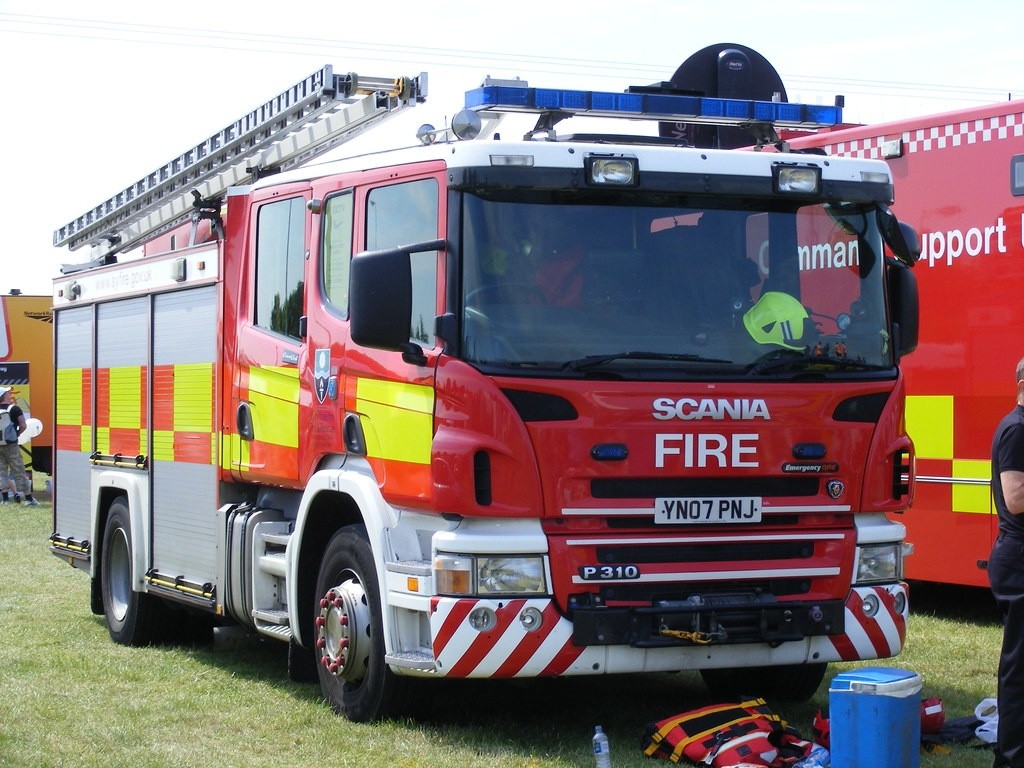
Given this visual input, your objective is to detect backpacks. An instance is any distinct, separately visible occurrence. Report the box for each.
[0,404,18,445]
[645,697,813,768]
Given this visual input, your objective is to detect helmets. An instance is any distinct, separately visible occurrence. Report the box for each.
[743,291,810,351]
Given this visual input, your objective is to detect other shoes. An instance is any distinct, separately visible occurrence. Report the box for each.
[25,497,40,506]
[2,500,9,505]
[14,494,21,504]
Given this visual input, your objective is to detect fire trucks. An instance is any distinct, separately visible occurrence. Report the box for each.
[653,100,1024,611]
[49,42,920,725]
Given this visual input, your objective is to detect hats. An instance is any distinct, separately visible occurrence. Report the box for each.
[0,387,11,397]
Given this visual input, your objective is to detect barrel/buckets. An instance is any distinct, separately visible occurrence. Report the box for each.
[829,666,922,768]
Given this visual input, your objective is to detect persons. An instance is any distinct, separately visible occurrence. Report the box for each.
[0,387,39,506]
[986,356,1024,768]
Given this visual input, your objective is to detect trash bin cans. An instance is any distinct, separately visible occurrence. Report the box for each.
[826,667,925,768]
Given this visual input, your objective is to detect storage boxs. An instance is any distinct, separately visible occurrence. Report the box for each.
[829,667,923,768]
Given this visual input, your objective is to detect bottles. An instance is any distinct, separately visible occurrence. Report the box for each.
[790,747,830,768]
[592,727,610,768]
